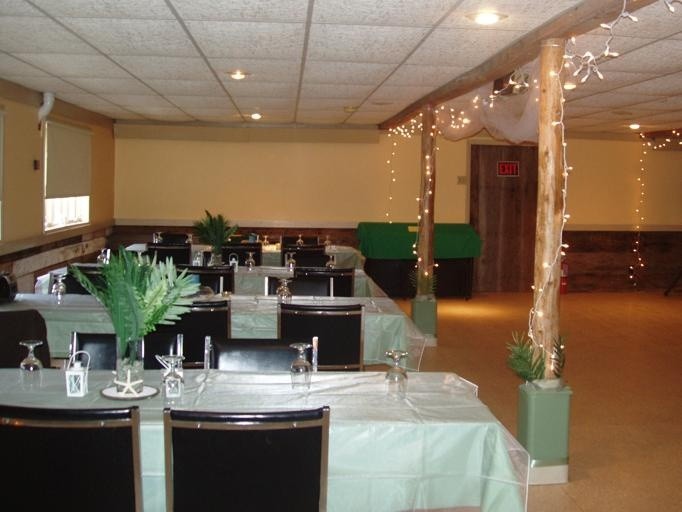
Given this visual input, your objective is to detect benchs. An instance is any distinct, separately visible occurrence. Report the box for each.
[565,250,647,291]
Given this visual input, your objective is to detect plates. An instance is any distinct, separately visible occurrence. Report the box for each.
[101,386,159,400]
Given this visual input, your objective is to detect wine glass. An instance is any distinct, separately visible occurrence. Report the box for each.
[288,342,313,389]
[51,275,69,306]
[96,230,336,271]
[17,339,45,388]
[162,355,187,406]
[382,350,408,401]
[276,278,292,305]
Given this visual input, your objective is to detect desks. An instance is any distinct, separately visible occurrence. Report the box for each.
[125,243,367,269]
[48,262,370,298]
[0,368,488,512]
[1,292,406,372]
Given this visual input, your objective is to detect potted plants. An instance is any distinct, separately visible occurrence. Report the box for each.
[188,212,238,267]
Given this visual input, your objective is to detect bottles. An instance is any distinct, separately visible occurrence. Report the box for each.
[64,360,88,398]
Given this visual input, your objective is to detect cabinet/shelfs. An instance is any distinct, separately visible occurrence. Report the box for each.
[360,221,482,301]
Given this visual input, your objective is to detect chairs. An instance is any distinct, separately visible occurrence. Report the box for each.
[280,236,335,266]
[176,264,235,295]
[1,309,59,370]
[164,405,330,511]
[65,264,114,293]
[203,333,320,373]
[222,236,263,264]
[277,302,365,372]
[65,244,206,335]
[264,274,335,297]
[147,232,193,265]
[140,301,233,369]
[3,405,144,511]
[69,329,185,367]
[290,265,358,295]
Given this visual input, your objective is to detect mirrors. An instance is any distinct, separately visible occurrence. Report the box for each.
[40,120,94,235]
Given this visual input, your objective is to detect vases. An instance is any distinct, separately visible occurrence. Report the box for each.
[115,335,143,394]
